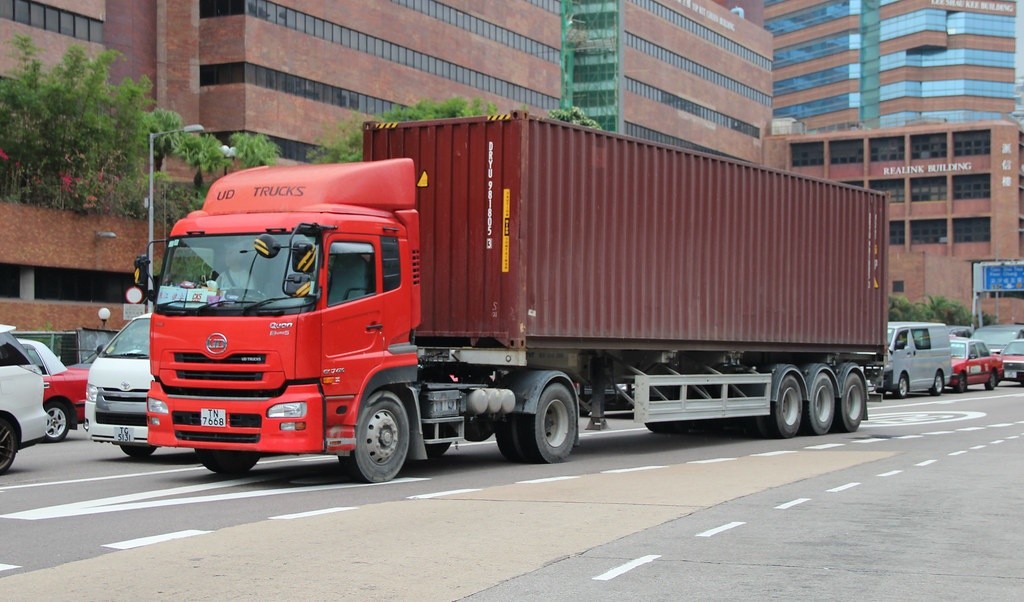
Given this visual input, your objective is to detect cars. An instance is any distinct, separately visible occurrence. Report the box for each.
[0,324,48,473]
[970,324,1024,354]
[84,312,157,459]
[17,338,98,443]
[947,325,974,339]
[998,339,1024,387]
[946,337,1003,393]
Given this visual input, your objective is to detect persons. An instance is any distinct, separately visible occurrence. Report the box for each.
[216,246,254,290]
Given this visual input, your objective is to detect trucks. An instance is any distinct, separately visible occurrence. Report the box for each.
[134,108,891,484]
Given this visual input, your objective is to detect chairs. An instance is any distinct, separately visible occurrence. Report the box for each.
[325,253,370,306]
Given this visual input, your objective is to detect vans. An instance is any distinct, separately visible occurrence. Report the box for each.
[861,322,952,399]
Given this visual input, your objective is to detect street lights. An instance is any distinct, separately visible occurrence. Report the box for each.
[148,123,204,312]
[219,145,237,176]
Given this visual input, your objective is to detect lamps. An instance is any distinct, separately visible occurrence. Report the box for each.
[97,231,116,239]
